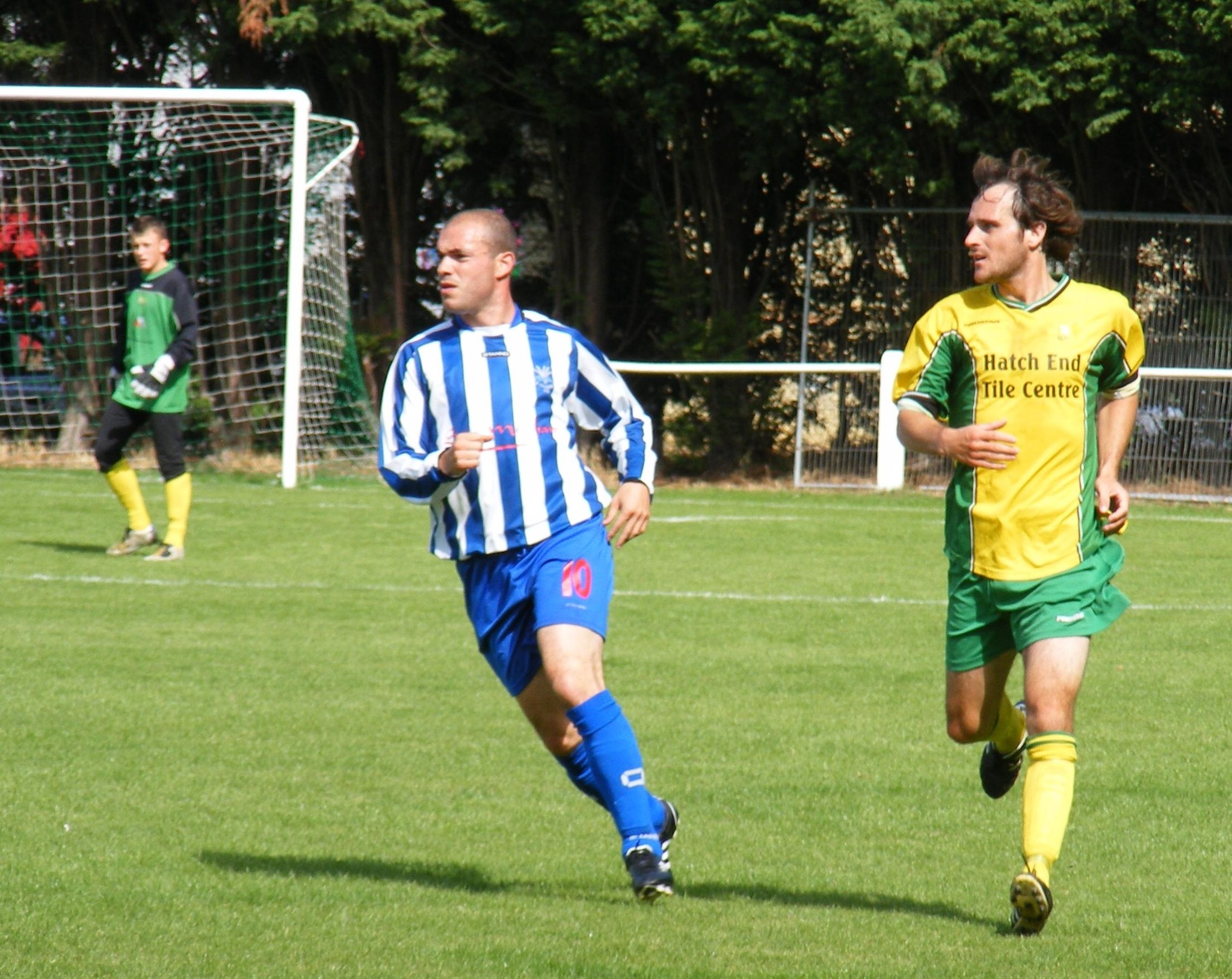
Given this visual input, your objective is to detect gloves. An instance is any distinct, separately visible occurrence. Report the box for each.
[130,353,175,399]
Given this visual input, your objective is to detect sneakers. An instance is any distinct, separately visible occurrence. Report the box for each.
[106,527,158,555]
[656,797,678,895]
[623,845,674,901]
[1009,848,1053,936]
[147,543,184,561]
[980,701,1029,798]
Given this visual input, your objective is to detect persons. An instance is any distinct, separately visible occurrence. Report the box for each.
[377,209,680,900]
[94,216,198,561]
[891,149,1146,937]
